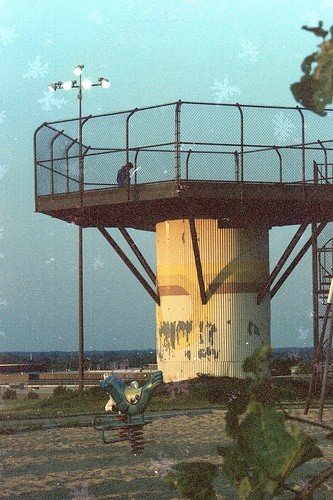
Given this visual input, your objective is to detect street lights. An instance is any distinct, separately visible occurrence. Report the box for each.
[48,65,112,389]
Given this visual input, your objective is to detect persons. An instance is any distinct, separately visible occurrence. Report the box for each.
[116,162,133,189]
